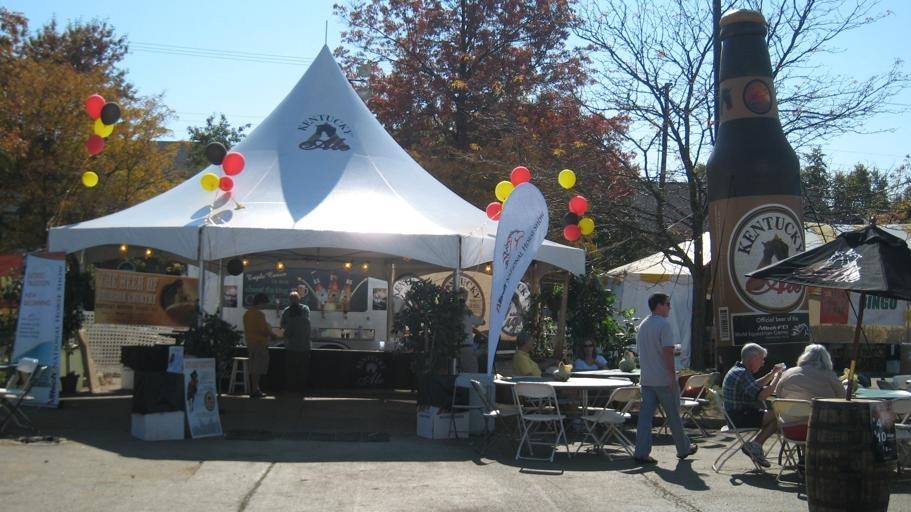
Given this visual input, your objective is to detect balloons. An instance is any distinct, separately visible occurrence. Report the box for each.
[511,166,531,187]
[95,118,114,138]
[564,213,577,224]
[83,171,98,187]
[202,174,220,191]
[559,169,576,188]
[87,135,104,155]
[486,202,502,220]
[564,225,581,241]
[86,94,105,119]
[222,153,245,175]
[220,177,233,191]
[207,142,227,164]
[569,196,588,216]
[101,103,121,124]
[578,218,594,235]
[495,180,515,202]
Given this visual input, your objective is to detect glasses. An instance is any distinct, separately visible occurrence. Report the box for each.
[584,344,593,347]
[666,302,670,306]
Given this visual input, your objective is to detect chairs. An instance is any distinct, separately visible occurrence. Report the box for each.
[708,387,765,476]
[772,398,812,487]
[575,387,639,463]
[659,373,719,440]
[577,375,630,413]
[515,381,571,463]
[448,372,492,442]
[470,379,523,454]
[0,357,47,433]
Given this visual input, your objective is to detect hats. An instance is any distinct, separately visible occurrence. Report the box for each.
[253,293,269,304]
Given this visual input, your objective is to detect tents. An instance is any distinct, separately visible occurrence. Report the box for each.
[598,222,911,370]
[47,42,584,375]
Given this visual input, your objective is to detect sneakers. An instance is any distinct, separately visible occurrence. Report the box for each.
[743,442,770,468]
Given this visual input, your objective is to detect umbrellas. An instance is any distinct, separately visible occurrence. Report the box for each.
[745,214,911,399]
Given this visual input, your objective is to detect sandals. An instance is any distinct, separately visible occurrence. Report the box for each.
[635,456,658,464]
[676,442,698,460]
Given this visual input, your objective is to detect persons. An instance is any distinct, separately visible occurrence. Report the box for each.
[283,305,312,397]
[775,343,847,440]
[574,337,609,369]
[722,342,783,467]
[634,293,697,464]
[242,293,270,399]
[280,291,310,349]
[513,333,542,377]
[190,298,199,331]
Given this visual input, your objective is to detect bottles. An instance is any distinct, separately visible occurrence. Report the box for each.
[328,271,339,310]
[340,274,354,312]
[309,269,327,310]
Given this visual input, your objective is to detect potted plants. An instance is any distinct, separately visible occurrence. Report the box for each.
[59,264,97,394]
[391,274,473,441]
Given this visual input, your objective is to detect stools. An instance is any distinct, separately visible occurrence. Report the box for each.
[228,356,249,395]
